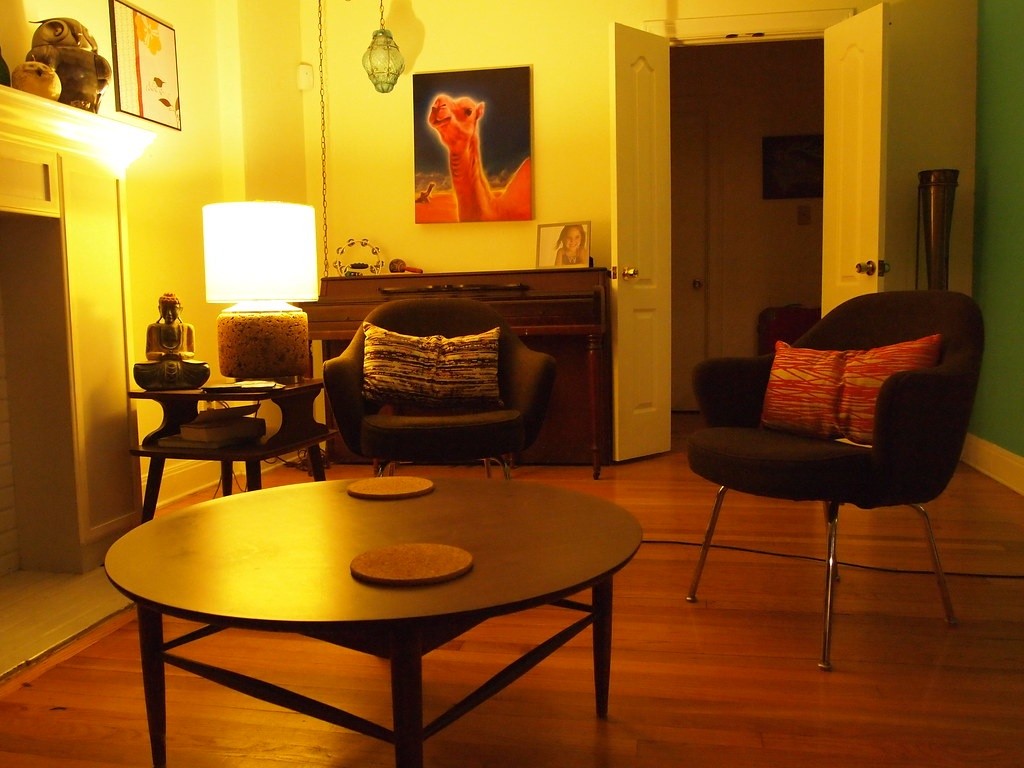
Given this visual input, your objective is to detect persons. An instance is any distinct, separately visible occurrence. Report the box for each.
[554,225,588,267]
[134,293,210,389]
[158,402,266,449]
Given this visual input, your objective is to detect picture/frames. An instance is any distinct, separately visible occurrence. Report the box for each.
[534,221,591,268]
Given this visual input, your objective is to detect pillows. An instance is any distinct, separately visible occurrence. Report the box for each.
[361,322,505,405]
[759,333,944,445]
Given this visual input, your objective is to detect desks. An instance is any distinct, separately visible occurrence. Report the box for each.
[104,477,644,768]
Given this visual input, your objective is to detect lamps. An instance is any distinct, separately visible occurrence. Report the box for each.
[362,0,405,93]
[202,200,319,386]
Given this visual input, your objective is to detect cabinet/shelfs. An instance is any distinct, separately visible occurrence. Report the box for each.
[124,375,337,526]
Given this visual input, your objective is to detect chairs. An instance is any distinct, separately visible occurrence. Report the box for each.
[684,289,986,670]
[323,298,558,478]
